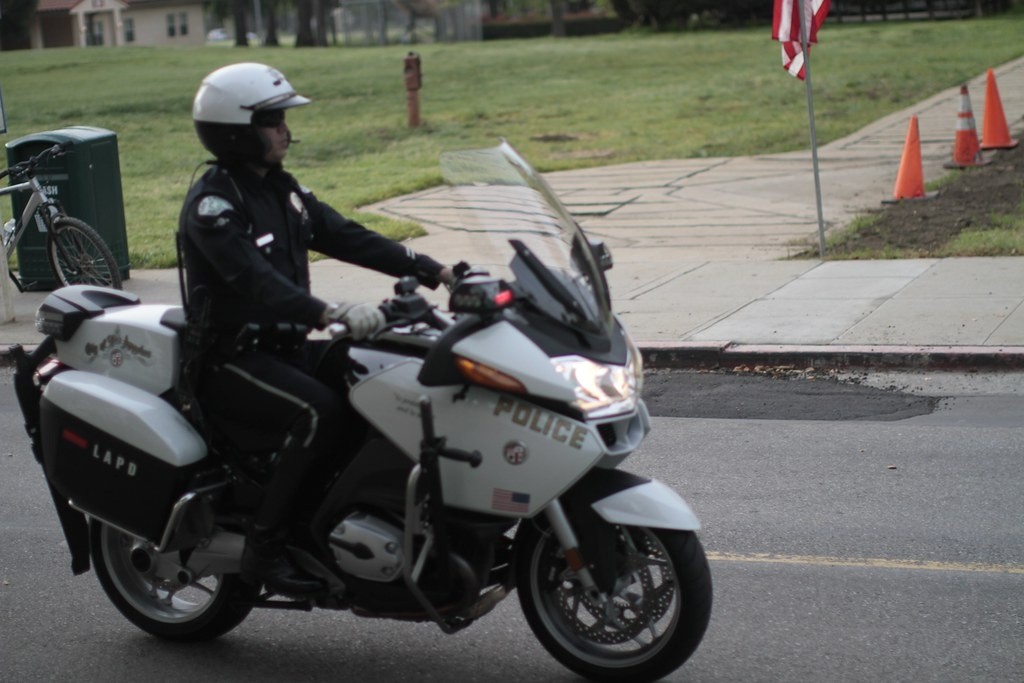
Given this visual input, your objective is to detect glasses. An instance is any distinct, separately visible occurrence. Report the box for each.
[242,109,289,129]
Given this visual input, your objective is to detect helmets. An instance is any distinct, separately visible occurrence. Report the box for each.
[190,62,314,123]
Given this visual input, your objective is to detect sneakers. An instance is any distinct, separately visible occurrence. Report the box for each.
[240,539,346,600]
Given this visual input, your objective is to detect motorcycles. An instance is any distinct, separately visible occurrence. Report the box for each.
[5,140,718,683]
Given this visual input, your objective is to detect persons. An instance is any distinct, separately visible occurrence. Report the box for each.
[174,61,474,595]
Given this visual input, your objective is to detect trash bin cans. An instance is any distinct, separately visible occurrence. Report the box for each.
[4,124,132,292]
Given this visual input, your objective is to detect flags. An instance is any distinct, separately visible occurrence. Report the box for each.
[772,1,831,79]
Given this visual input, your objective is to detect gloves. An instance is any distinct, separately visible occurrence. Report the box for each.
[435,266,482,298]
[318,300,389,344]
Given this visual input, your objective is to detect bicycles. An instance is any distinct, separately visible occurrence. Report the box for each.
[0,141,123,299]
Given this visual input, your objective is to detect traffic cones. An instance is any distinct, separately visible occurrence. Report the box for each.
[942,81,993,169]
[886,113,936,204]
[973,67,1019,152]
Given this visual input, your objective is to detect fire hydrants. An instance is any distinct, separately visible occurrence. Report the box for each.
[401,48,425,128]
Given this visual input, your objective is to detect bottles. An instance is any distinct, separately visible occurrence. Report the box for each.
[2,218,15,246]
